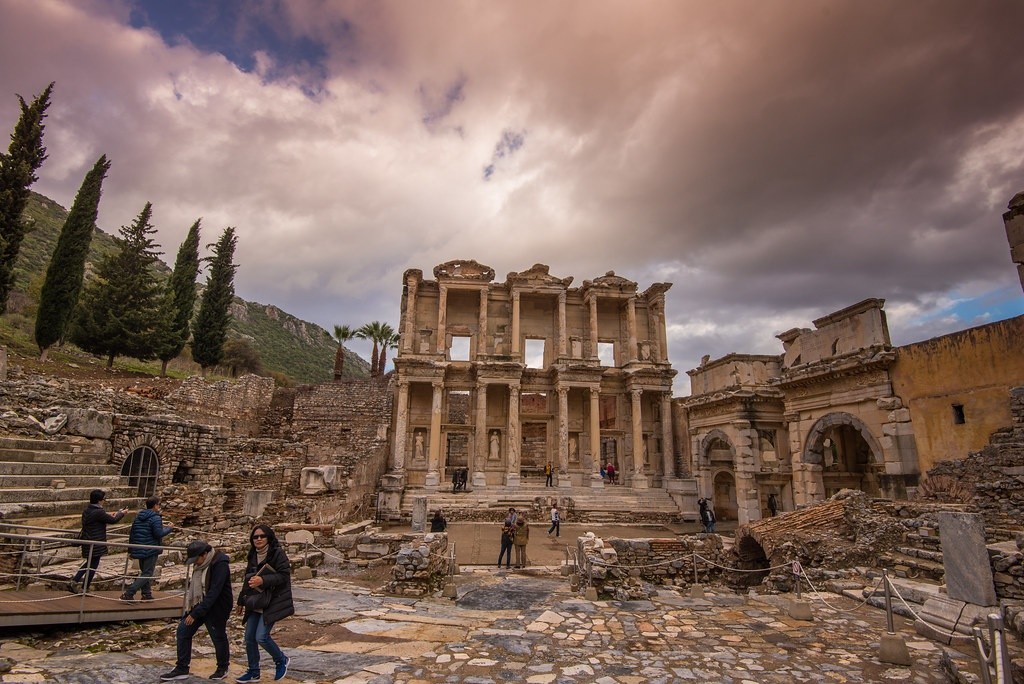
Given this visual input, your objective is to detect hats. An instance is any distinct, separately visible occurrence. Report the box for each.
[516,516,525,524]
[185,542,208,565]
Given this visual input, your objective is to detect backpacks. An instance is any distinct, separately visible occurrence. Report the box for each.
[502,528,511,545]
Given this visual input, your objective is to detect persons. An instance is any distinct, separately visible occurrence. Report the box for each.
[452,467,469,494]
[768,494,777,516]
[415,432,424,459]
[569,438,576,460]
[430,511,446,532]
[544,461,553,487]
[547,503,559,537]
[698,497,716,532]
[161,541,233,681]
[69,489,128,597]
[498,508,529,568]
[119,496,173,604]
[236,525,294,683]
[600,463,615,484]
[490,431,499,459]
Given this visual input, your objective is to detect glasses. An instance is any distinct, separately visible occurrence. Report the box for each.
[252,534,267,539]
[100,499,105,501]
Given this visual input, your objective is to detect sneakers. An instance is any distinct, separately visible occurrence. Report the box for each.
[274,656,290,681]
[209,668,228,680]
[160,666,189,681]
[235,669,260,683]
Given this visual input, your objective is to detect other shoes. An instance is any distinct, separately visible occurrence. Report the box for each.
[78,588,94,595]
[140,596,155,603]
[506,566,511,569]
[498,565,501,568]
[557,536,562,537]
[119,593,140,605]
[514,566,520,568]
[67,583,77,594]
[550,485,553,487]
[546,485,548,487]
[547,531,550,536]
[523,566,525,568]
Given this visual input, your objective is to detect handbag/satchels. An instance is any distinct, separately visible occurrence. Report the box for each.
[71,536,81,547]
[244,588,272,610]
